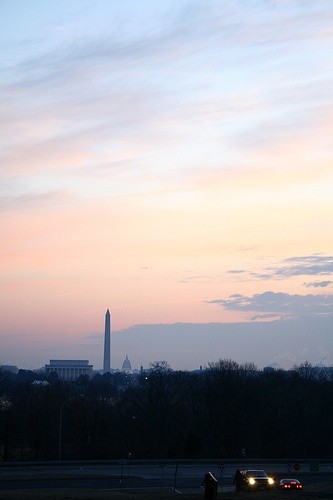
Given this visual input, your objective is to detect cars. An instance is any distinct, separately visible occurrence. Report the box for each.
[239,470,275,492]
[277,479,303,493]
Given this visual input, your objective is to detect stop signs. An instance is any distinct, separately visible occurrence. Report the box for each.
[217,462,225,469]
